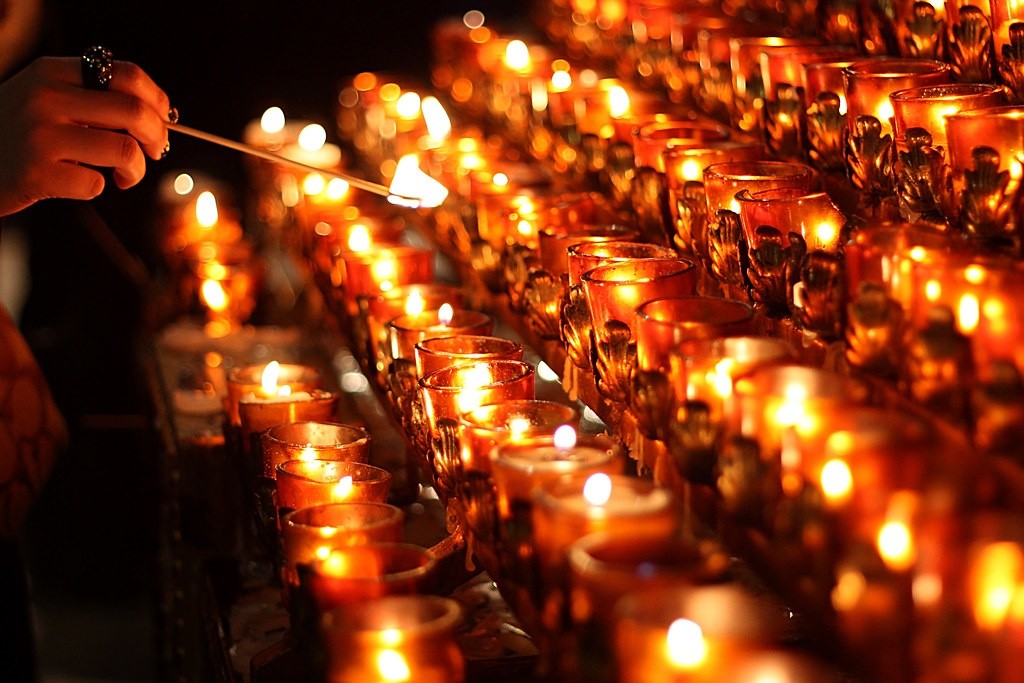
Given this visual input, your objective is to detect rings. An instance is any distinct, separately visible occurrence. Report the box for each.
[79,45,112,90]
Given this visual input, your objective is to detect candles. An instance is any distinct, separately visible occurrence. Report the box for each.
[159,0,1024,683]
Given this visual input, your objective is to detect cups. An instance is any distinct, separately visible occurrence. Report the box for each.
[136,0,1024,683]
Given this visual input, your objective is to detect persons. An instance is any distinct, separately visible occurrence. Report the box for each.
[0,0,179,683]
